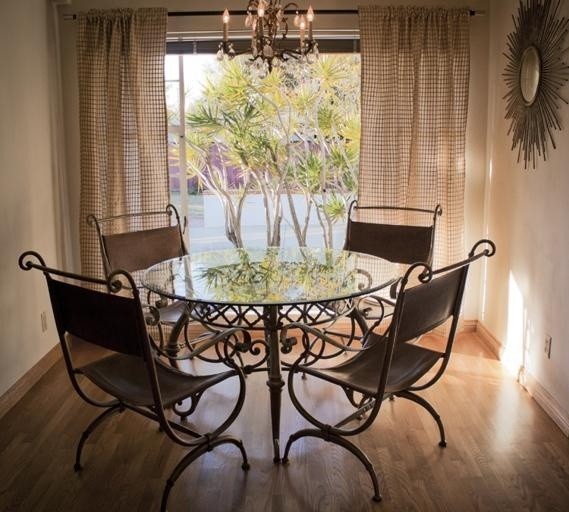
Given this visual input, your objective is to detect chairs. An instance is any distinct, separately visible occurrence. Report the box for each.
[82,203,245,405]
[20,251,249,511]
[281,238,497,502]
[299,199,443,418]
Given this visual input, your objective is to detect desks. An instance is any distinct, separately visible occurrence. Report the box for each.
[141,248,403,464]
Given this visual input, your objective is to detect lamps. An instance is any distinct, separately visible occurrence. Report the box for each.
[218,0,322,72]
